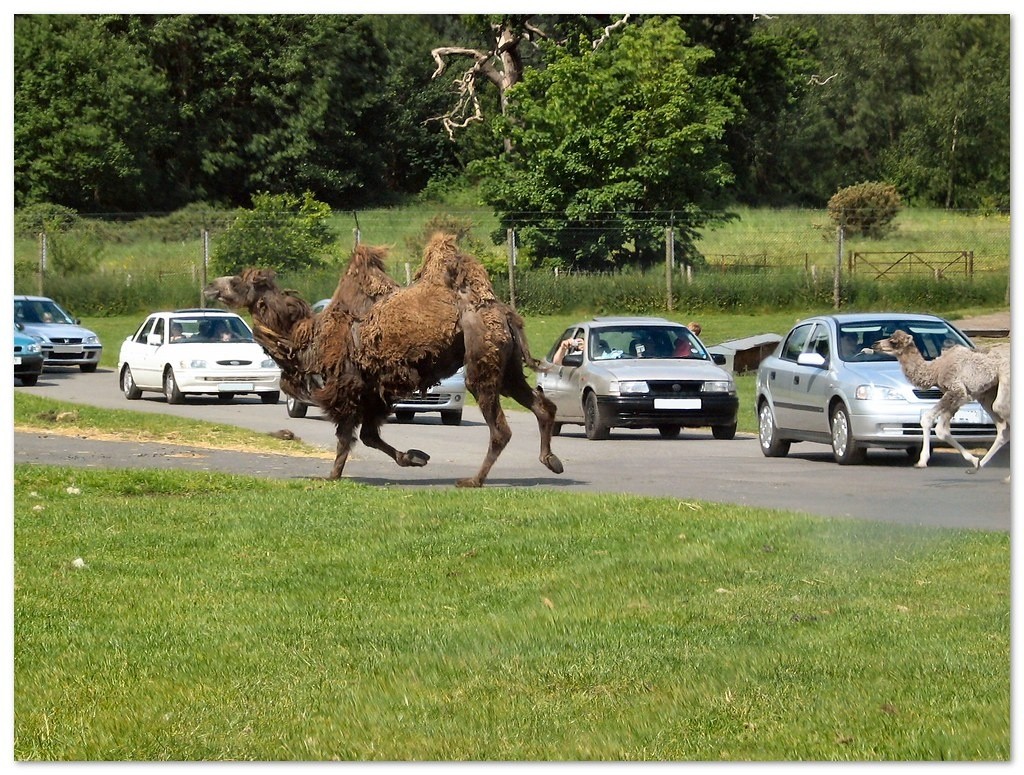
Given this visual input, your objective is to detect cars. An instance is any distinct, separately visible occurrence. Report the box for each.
[537,316,738,440]
[14,324,43,383]
[118,310,283,404]
[14,296,102,373]
[285,298,467,423]
[754,313,999,465]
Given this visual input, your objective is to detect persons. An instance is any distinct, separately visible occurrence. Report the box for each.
[839,332,873,358]
[41,312,53,323]
[171,323,186,341]
[220,329,232,341]
[674,322,701,357]
[552,337,611,369]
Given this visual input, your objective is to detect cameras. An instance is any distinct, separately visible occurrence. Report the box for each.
[571,340,579,347]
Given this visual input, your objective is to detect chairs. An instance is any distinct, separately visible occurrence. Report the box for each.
[630,339,655,358]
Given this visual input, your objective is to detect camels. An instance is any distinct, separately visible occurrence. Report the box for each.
[871,329,1010,485]
[205,233,564,487]
